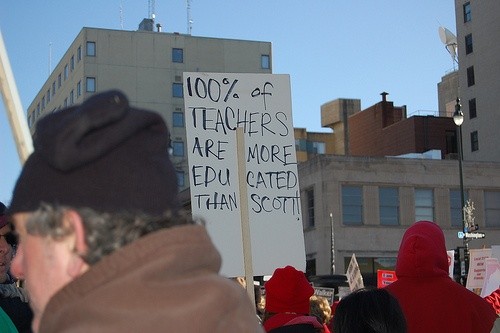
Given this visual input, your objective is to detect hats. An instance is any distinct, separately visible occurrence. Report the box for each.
[265,265,315,315]
[0,202,9,228]
[7,88,182,213]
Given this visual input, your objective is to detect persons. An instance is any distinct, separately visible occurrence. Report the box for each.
[332,287,407,333]
[8,88,264,333]
[380,221,500,333]
[0,202,35,333]
[254,266,332,333]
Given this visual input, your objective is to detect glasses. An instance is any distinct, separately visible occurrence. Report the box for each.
[0,231,17,245]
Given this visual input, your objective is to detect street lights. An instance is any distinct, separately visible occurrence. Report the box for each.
[453,96,469,273]
